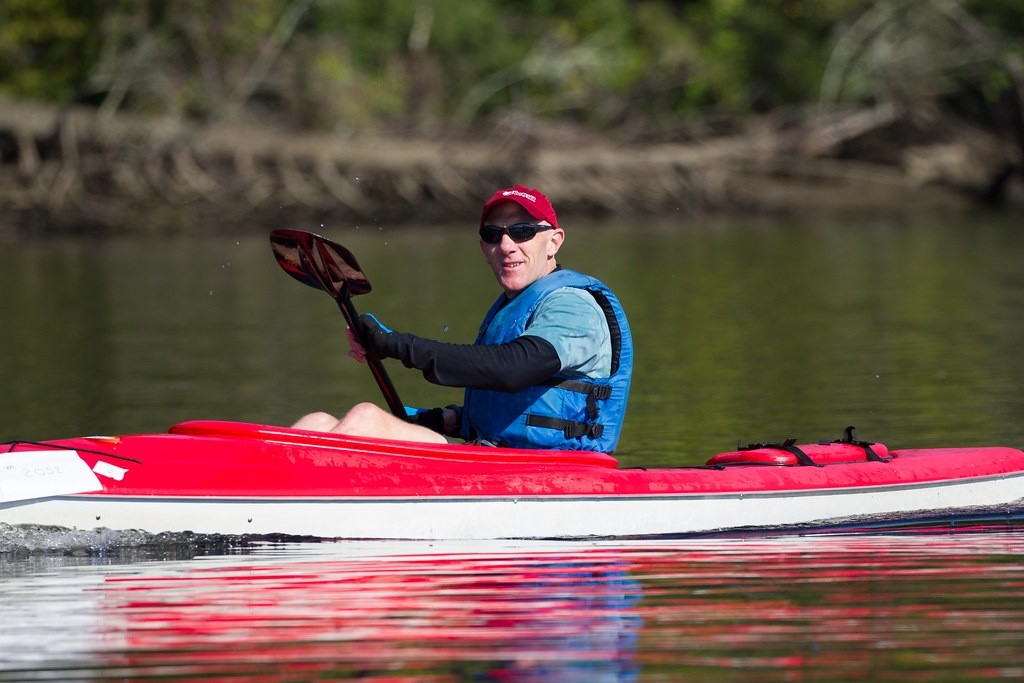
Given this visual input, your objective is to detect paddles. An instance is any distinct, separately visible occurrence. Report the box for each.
[265,225,417,423]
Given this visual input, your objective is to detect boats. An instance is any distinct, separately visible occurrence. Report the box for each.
[0,420,1024,546]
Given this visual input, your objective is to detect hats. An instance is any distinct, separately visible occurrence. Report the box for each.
[480,184,559,228]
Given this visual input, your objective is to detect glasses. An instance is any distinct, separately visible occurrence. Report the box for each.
[479,222,553,244]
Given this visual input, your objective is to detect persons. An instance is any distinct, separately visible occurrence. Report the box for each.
[286,183,634,456]
